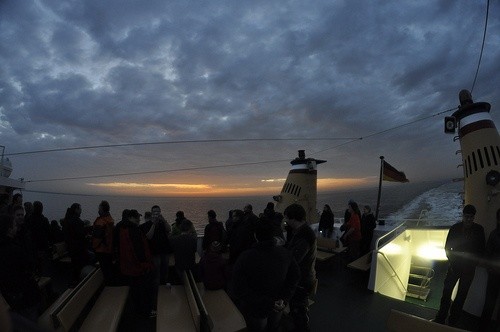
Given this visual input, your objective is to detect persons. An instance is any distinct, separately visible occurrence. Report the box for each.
[0,193,376,332]
[480,209,500,332]
[429,204,486,327]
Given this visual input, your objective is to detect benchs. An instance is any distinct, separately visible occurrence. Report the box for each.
[34,267,128,332]
[155,269,247,332]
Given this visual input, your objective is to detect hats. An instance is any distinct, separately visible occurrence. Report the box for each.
[128,210,142,218]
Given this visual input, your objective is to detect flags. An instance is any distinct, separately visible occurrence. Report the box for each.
[380,159,409,183]
